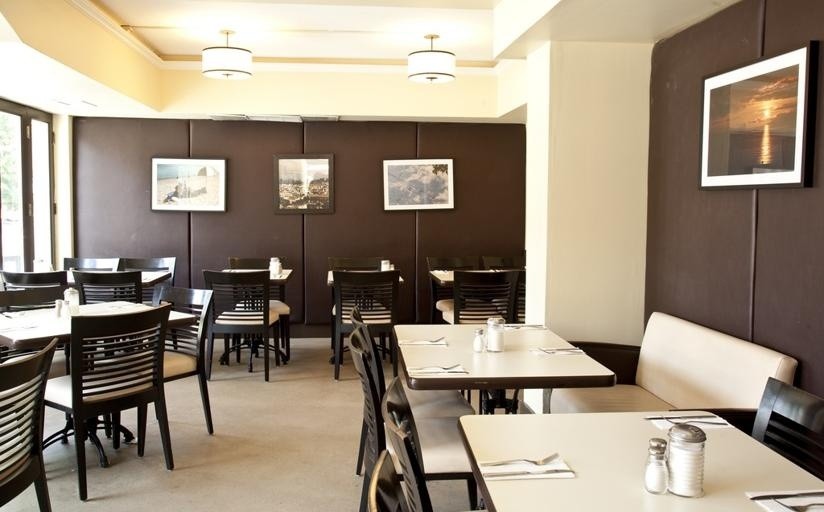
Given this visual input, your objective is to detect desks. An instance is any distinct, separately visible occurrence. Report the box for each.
[1,300,197,468]
[392,324,617,414]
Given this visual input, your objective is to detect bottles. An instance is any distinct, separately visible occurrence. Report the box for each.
[472,316,506,352]
[266,256,284,277]
[380,260,393,272]
[53,287,81,318]
[646,425,705,496]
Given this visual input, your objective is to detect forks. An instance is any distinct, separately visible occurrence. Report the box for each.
[481,454,562,466]
[773,498,824,512]
[398,336,449,343]
[660,417,728,426]
[406,364,461,370]
[540,350,585,355]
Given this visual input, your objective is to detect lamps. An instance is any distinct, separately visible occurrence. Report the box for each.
[200,27,253,82]
[407,33,456,84]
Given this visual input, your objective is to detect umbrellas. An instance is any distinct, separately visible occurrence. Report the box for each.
[198,165,218,191]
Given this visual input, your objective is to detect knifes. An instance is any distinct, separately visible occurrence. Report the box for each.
[508,324,546,331]
[750,491,822,501]
[643,415,719,420]
[535,347,580,350]
[398,343,448,346]
[483,469,575,478]
[408,371,472,376]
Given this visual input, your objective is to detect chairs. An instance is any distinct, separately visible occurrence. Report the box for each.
[1,336,59,512]
[110,285,215,457]
[382,375,478,512]
[751,377,823,480]
[366,449,411,511]
[425,253,525,324]
[457,411,823,511]
[44,303,175,503]
[348,325,479,511]
[200,256,297,383]
[2,247,178,317]
[326,254,405,381]
[349,305,475,476]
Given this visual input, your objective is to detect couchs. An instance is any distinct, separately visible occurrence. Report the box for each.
[521,311,797,414]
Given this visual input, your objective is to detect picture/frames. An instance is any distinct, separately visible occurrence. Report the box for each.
[378,156,457,212]
[697,39,816,189]
[149,154,229,213]
[273,152,335,215]
